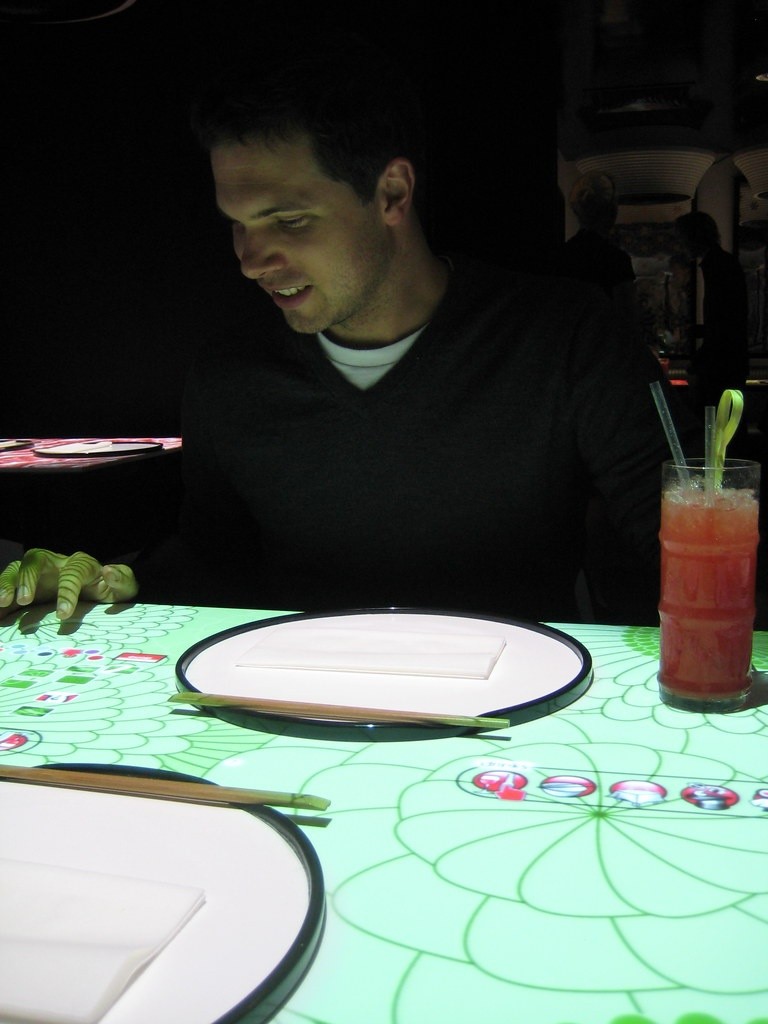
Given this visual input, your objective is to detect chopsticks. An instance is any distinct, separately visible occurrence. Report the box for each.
[0,764,331,811]
[168,692,510,728]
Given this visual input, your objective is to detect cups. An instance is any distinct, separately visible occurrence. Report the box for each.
[659,458,761,713]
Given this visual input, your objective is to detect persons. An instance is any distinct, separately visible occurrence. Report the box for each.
[0,28,681,620]
[551,161,748,432]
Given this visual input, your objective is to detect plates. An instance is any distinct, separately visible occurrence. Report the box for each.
[0,764,327,1024]
[176,604,593,745]
[0,440,34,452]
[32,442,163,457]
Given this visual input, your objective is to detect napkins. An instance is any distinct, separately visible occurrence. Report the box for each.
[0,858,205,1024]
[236,637,507,679]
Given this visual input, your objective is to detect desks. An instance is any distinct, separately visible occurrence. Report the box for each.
[0,437,184,557]
[0,607,768,1024]
